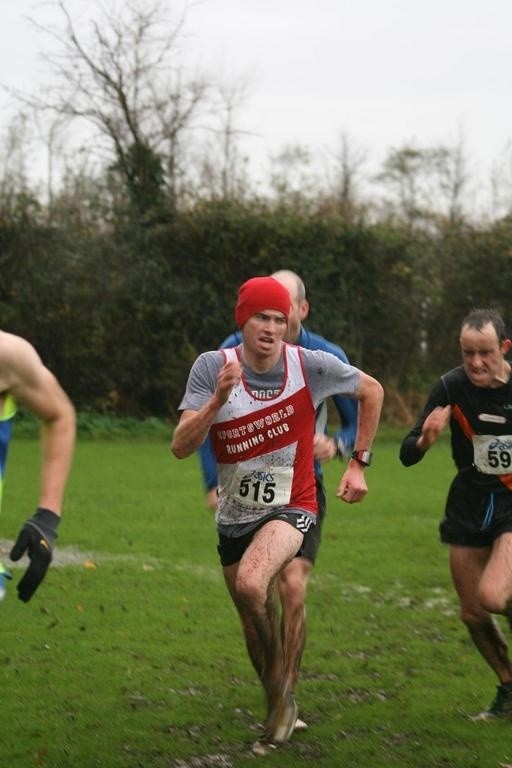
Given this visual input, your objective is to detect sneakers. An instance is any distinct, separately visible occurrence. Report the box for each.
[484,682,512,717]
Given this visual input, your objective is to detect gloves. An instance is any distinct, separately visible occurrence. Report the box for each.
[10,507,60,603]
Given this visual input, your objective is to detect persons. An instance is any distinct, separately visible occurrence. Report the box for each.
[0,329,77,602]
[400,309,512,719]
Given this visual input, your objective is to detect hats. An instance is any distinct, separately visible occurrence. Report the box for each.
[236,276,292,334]
